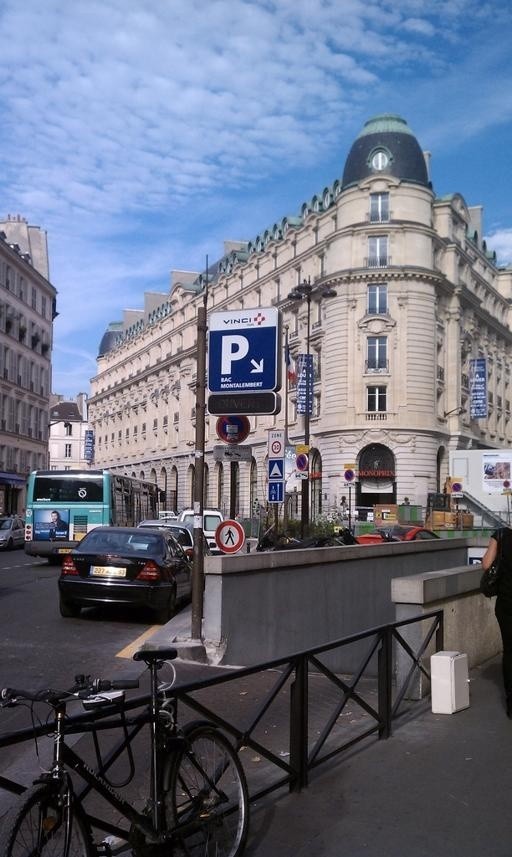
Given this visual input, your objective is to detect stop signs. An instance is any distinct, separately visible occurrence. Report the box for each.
[344,469,355,482]
[503,481,510,488]
[452,482,462,493]
[295,454,309,472]
[215,415,250,444]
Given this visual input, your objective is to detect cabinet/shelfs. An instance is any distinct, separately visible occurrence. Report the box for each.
[430,650,471,714]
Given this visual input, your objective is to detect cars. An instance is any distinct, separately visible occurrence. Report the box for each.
[355,524,443,544]
[159,510,179,523]
[57,526,193,624]
[0,517,25,551]
[127,519,216,564]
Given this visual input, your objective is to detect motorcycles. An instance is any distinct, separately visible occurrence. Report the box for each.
[255,525,361,554]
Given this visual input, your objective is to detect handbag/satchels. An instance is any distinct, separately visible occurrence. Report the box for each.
[480,565,500,598]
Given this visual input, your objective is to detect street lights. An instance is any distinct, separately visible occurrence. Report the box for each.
[287,274,337,529]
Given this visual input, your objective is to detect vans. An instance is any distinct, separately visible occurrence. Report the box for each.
[177,507,225,554]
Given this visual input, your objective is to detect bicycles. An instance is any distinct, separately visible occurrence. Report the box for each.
[0,646,251,857]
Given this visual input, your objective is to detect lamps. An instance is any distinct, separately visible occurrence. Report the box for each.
[47,419,71,428]
[444,406,467,417]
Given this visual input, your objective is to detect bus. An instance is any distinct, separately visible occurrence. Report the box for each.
[24,468,166,564]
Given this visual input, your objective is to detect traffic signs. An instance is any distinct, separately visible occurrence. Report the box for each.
[295,352,313,414]
[468,357,487,418]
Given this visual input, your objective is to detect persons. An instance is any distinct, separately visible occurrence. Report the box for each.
[402,497,410,505]
[480,526,512,719]
[443,475,453,509]
[48,511,68,543]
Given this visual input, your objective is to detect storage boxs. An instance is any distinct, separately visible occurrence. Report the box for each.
[426,493,474,528]
